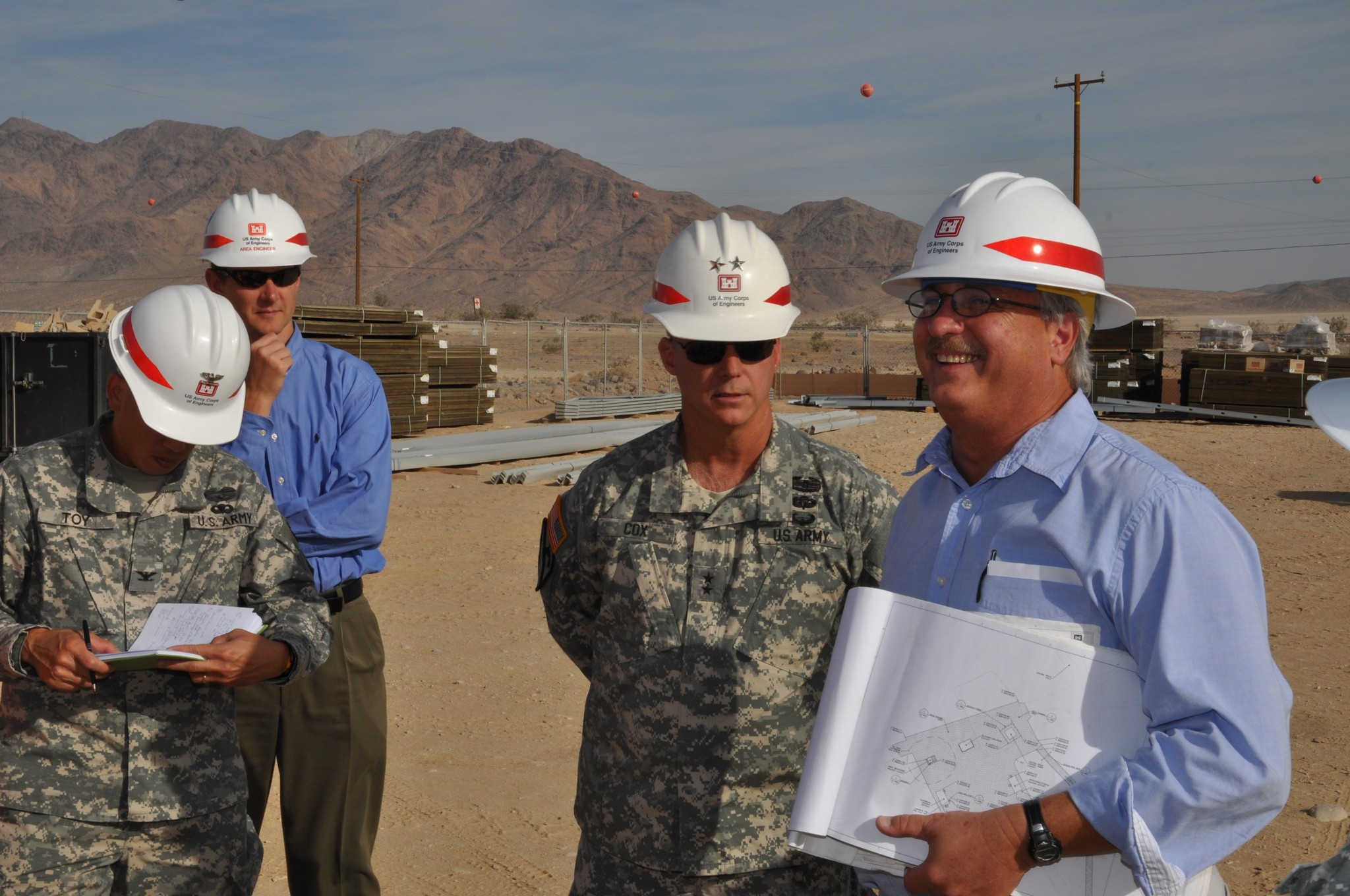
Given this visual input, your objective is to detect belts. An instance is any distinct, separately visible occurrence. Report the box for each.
[321,576,363,613]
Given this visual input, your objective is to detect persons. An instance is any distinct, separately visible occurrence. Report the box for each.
[200,188,391,896]
[538,212,898,896]
[880,171,1293,896]
[0,285,332,896]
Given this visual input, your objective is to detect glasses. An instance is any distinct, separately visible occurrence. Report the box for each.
[211,263,301,288]
[905,286,1044,318]
[672,339,777,365]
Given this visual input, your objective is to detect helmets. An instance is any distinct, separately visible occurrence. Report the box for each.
[881,171,1136,330]
[1305,376,1349,449]
[197,187,318,268]
[110,283,249,445]
[644,211,802,342]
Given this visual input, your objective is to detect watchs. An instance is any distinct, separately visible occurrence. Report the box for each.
[283,650,295,677]
[1023,798,1063,866]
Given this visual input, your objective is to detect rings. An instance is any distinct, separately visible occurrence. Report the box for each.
[202,674,208,684]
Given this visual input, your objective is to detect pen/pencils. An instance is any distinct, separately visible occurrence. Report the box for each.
[976,549,997,603]
[82,619,97,692]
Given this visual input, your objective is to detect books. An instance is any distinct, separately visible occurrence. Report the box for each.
[788,587,1153,896]
[95,602,268,671]
